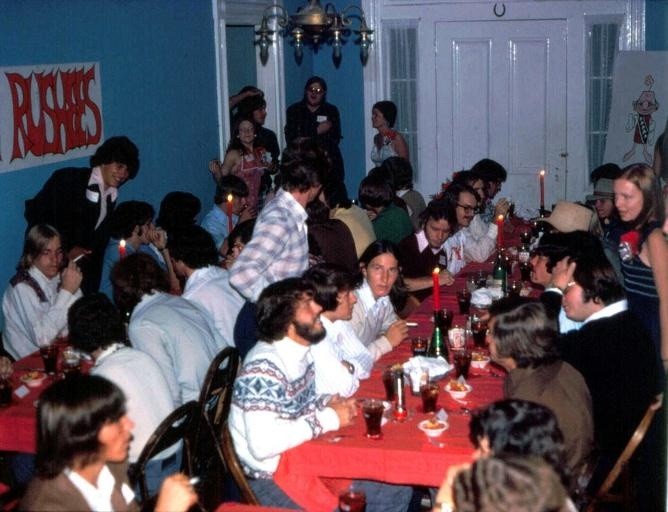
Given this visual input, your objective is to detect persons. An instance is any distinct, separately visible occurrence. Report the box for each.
[540,227,665,444]
[439,180,481,276]
[23,135,140,295]
[370,101,410,166]
[301,263,374,398]
[470,159,507,224]
[613,162,667,383]
[528,231,561,289]
[486,296,597,493]
[225,218,256,269]
[208,116,269,217]
[97,200,182,308]
[19,374,198,511]
[225,276,413,512]
[368,167,409,216]
[431,452,571,511]
[165,224,248,377]
[661,184,668,235]
[584,178,629,248]
[317,175,379,266]
[238,95,281,215]
[229,134,335,365]
[653,118,668,178]
[154,191,202,293]
[358,177,414,244]
[1,357,14,380]
[453,170,511,265]
[200,175,257,267]
[228,85,265,133]
[591,163,622,191]
[468,398,585,511]
[11,291,186,504]
[305,197,359,274]
[347,239,410,365]
[380,156,427,232]
[1,224,84,361]
[110,251,230,422]
[622,73,659,163]
[397,198,458,301]
[284,76,345,181]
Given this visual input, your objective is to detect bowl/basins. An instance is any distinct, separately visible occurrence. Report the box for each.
[417,418,448,436]
[472,353,490,369]
[21,372,46,387]
[444,383,473,399]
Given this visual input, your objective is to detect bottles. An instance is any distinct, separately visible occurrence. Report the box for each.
[493,249,507,294]
[428,312,450,362]
[539,209,548,238]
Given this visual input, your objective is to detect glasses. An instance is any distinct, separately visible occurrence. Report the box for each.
[306,85,322,94]
[562,280,578,296]
[239,125,255,133]
[455,202,482,215]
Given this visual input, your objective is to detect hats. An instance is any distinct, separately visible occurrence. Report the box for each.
[532,201,595,233]
[586,178,617,202]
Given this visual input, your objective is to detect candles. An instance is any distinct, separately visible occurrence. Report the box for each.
[433,267,440,314]
[119,239,126,258]
[227,194,232,232]
[540,170,545,206]
[497,215,502,247]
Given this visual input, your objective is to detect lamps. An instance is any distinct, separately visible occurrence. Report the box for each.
[255,0,375,69]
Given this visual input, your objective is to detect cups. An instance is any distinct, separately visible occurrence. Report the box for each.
[440,309,453,336]
[473,322,486,347]
[458,290,472,313]
[339,488,366,511]
[412,336,428,357]
[362,398,384,439]
[421,384,439,415]
[454,351,472,379]
[40,344,58,376]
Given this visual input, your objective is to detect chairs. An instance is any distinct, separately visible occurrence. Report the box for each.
[129,400,209,512]
[196,345,239,442]
[581,393,663,512]
[220,422,260,505]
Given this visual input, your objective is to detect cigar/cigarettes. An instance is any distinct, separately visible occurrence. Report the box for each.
[72,253,85,263]
[406,322,418,326]
[189,476,200,484]
